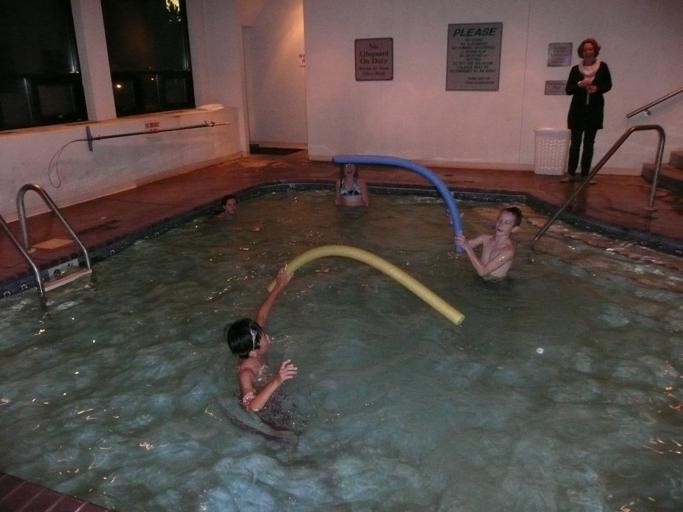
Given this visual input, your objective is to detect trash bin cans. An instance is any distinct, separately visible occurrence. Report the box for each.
[533,127,571,175]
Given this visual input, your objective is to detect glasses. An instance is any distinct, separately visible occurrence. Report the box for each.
[249,319,259,352]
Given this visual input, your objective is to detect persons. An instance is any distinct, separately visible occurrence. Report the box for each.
[560,38,611,183]
[331,165,370,209]
[226,263,301,429]
[216,196,265,232]
[454,207,521,282]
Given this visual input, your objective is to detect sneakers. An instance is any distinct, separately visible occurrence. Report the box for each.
[561,172,576,181]
[579,177,596,185]
